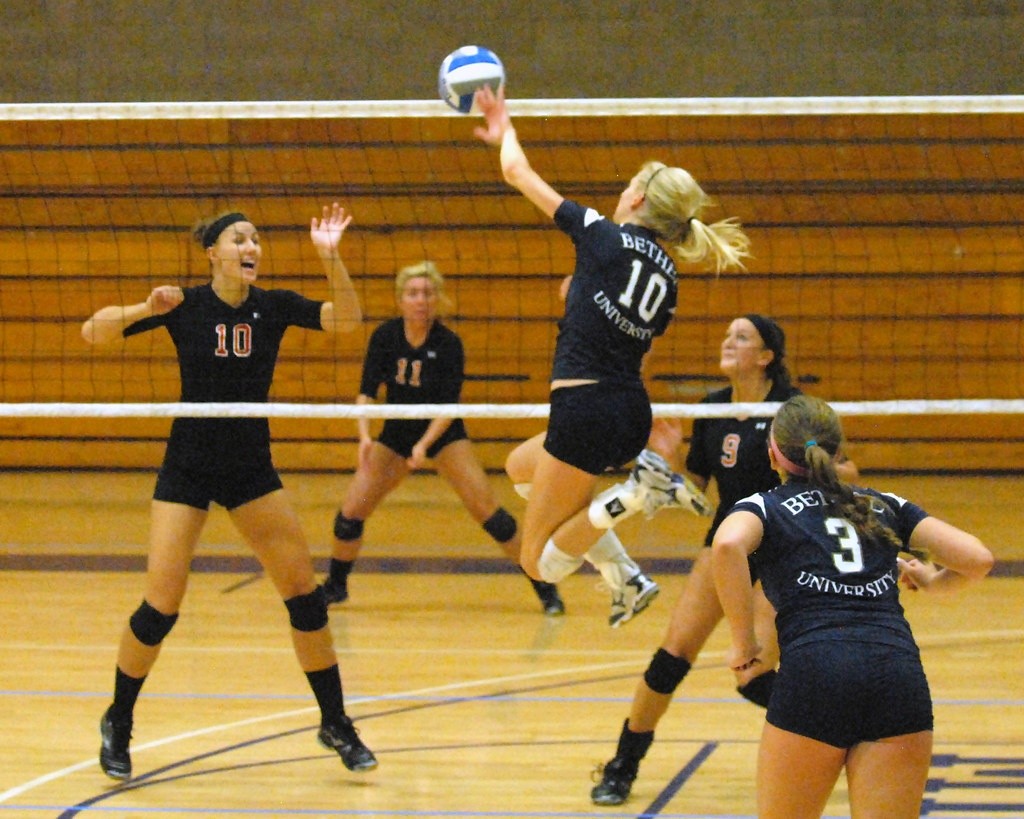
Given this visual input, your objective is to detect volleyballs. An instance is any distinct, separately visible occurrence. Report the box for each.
[435,43,509,115]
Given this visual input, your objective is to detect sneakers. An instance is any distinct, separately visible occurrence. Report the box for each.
[631,447,714,519]
[317,714,379,771]
[590,759,637,806]
[318,585,348,604]
[608,562,659,627]
[100,706,132,781]
[532,578,564,616]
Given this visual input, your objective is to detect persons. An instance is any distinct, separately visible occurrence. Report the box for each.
[472,82,753,630]
[321,261,565,617]
[710,393,994,819]
[590,312,858,806]
[80,201,379,781]
